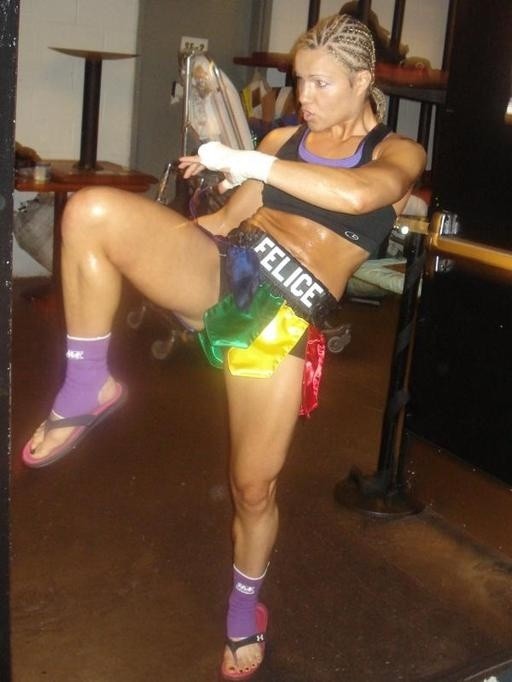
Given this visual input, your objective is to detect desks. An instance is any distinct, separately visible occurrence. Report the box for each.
[13,161,150,309]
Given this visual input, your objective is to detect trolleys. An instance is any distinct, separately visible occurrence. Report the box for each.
[129,161,354,361]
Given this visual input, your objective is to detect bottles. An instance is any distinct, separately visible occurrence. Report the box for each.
[34,161,52,182]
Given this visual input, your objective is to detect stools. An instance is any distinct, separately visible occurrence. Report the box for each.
[48,42,158,182]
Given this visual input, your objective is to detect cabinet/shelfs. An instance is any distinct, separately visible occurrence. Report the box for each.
[233,0,454,297]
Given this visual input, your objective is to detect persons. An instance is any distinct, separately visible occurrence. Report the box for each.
[18,8,432,681]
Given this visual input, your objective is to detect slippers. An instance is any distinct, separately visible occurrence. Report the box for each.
[220,602,268,680]
[22,375,129,470]
